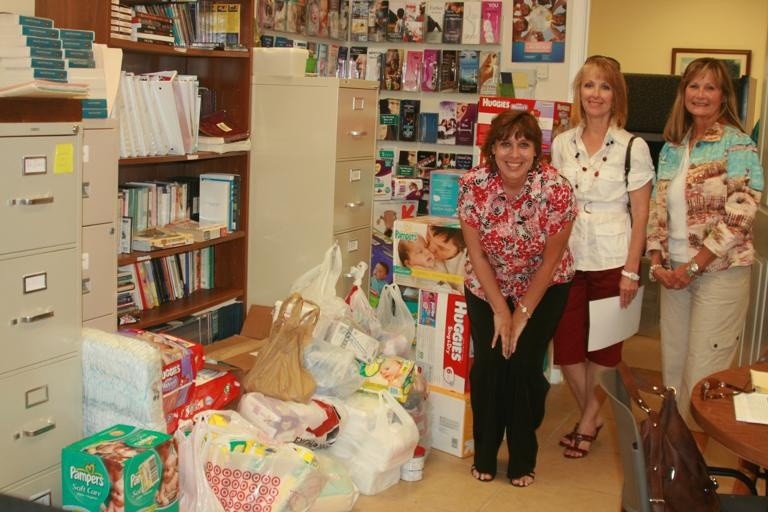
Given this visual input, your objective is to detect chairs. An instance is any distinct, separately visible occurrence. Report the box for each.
[593,382,768,512]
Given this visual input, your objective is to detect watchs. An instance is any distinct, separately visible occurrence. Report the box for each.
[688,257,702,277]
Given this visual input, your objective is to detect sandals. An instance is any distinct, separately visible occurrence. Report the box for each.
[471,464,494,481]
[511,471,535,487]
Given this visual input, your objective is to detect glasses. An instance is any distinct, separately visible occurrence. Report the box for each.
[701,378,755,401]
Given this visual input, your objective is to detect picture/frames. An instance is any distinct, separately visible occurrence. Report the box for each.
[671,48,752,77]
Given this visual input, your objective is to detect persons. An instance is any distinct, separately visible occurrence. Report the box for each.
[644,59,765,457]
[103,477,124,511]
[369,97,480,323]
[256,1,566,92]
[548,56,657,460]
[456,109,579,490]
[156,452,180,508]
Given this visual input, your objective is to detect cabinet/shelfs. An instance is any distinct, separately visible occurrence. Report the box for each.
[80,118,118,331]
[0,121,81,512]
[0,0,252,330]
[248,74,382,316]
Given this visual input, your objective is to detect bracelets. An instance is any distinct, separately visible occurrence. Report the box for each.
[517,301,531,321]
[649,264,662,282]
[621,270,640,282]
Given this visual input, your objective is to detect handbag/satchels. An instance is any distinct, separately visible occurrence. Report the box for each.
[640,390,720,512]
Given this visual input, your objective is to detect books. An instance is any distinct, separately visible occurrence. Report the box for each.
[116,71,249,343]
[111,1,249,52]
[1,13,124,119]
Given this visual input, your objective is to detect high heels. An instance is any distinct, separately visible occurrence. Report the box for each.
[560,423,602,446]
[564,432,593,458]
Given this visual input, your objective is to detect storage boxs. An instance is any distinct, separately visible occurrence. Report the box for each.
[64,425,180,512]
[418,289,474,393]
[203,305,273,410]
[429,384,476,459]
[392,214,466,296]
[430,169,468,218]
[475,95,574,152]
